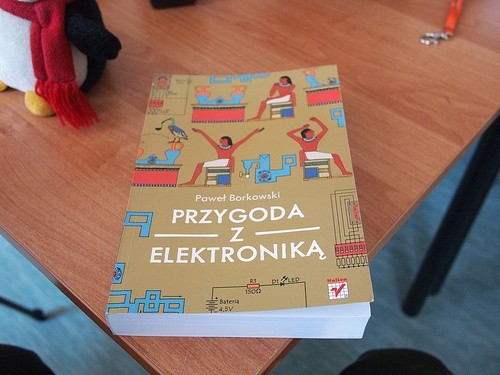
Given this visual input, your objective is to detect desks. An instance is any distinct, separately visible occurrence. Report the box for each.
[0,1,500,375]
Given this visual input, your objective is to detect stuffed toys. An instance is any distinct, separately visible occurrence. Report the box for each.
[0,0,121,130]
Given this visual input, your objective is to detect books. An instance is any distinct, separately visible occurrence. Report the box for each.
[104,64,373,339]
